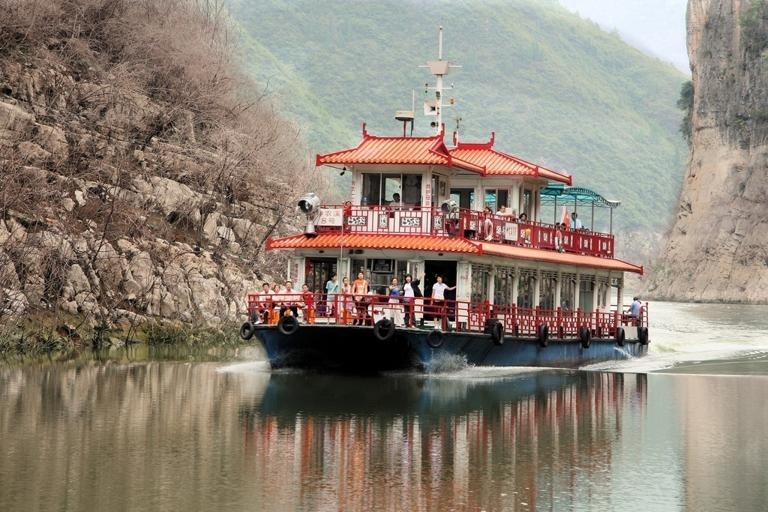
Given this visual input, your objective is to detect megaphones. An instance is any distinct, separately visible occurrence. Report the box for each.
[422,98,439,116]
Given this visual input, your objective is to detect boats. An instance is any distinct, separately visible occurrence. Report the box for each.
[239,22,650,373]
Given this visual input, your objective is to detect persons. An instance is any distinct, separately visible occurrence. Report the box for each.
[399,272,426,328]
[340,277,353,318]
[639,300,644,327]
[472,204,529,246]
[324,272,340,319]
[301,284,316,324]
[351,272,368,326]
[625,297,641,326]
[261,422,501,512]
[252,283,275,325]
[552,213,583,255]
[387,278,405,327]
[429,274,458,329]
[272,285,282,309]
[388,192,406,218]
[278,281,299,322]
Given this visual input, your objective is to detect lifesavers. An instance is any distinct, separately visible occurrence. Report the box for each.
[484,219,493,240]
[555,230,562,250]
